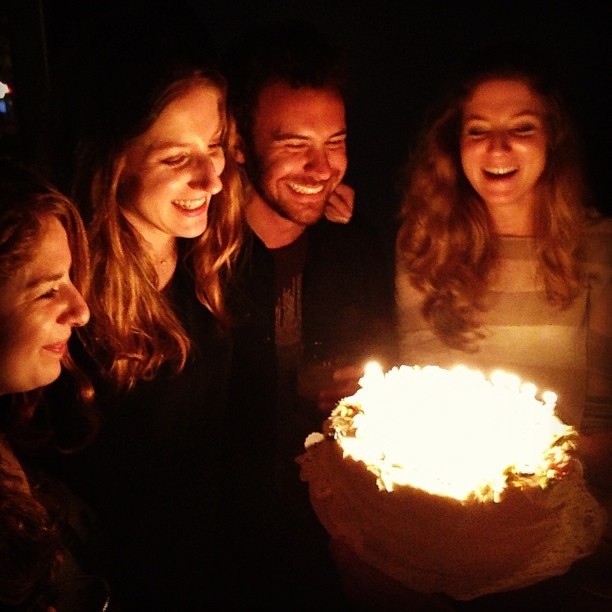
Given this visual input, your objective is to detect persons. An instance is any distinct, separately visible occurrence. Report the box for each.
[395,68,612,468]
[172,66,366,415]
[0,179,91,612]
[71,69,246,388]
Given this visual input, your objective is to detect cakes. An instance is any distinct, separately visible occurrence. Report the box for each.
[295,360,607,602]
